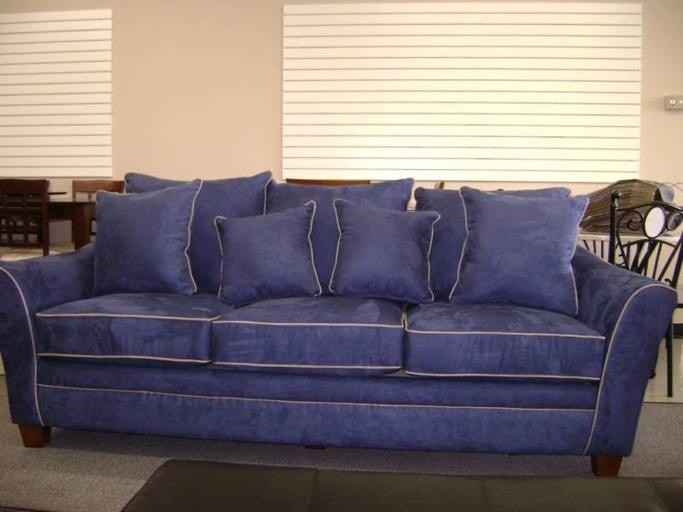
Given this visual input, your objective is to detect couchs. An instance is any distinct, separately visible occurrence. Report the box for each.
[0,242,677,478]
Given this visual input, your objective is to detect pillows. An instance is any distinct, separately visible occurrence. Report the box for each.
[328,199,442,304]
[98,179,205,295]
[124,171,272,293]
[449,187,589,316]
[215,201,322,307]
[413,186,571,299]
[266,177,414,290]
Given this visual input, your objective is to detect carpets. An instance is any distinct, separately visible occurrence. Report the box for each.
[0,374,683,511]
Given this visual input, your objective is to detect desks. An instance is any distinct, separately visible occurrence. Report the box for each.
[577,233,679,280]
[115,461,683,512]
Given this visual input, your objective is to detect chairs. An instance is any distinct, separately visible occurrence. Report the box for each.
[0,176,127,262]
[602,189,683,397]
